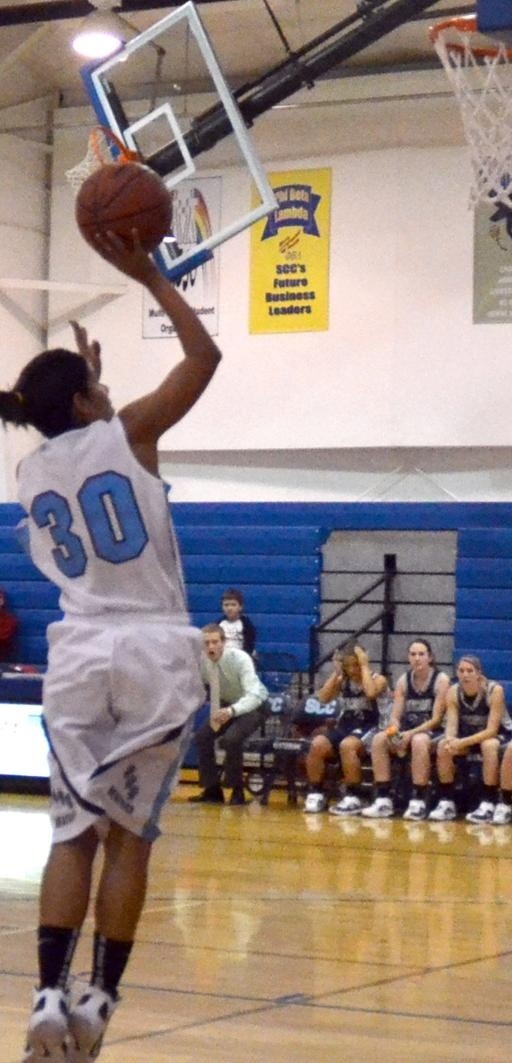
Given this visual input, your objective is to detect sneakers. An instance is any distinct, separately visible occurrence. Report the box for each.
[22,986,70,1063]
[302,793,330,814]
[329,796,363,816]
[465,801,495,824]
[428,801,456,821]
[402,799,427,822]
[361,797,395,818]
[71,986,116,1063]
[493,803,512,825]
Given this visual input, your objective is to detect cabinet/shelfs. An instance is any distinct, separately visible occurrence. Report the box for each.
[0,661,51,794]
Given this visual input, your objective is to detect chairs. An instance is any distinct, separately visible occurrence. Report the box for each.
[391,756,468,813]
[263,694,341,810]
[218,692,290,805]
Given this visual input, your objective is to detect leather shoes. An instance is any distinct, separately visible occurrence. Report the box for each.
[188,788,224,804]
[229,791,245,805]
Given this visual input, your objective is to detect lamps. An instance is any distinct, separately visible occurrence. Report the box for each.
[70,0,124,61]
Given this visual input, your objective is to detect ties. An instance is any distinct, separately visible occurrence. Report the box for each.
[210,664,220,734]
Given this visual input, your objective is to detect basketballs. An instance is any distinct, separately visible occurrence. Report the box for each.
[74,162,173,257]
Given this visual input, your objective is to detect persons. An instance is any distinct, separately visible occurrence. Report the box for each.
[432,654,511,818]
[216,589,255,660]
[306,644,389,812]
[360,639,448,822]
[0,227,219,1061]
[190,622,272,804]
[492,737,511,825]
